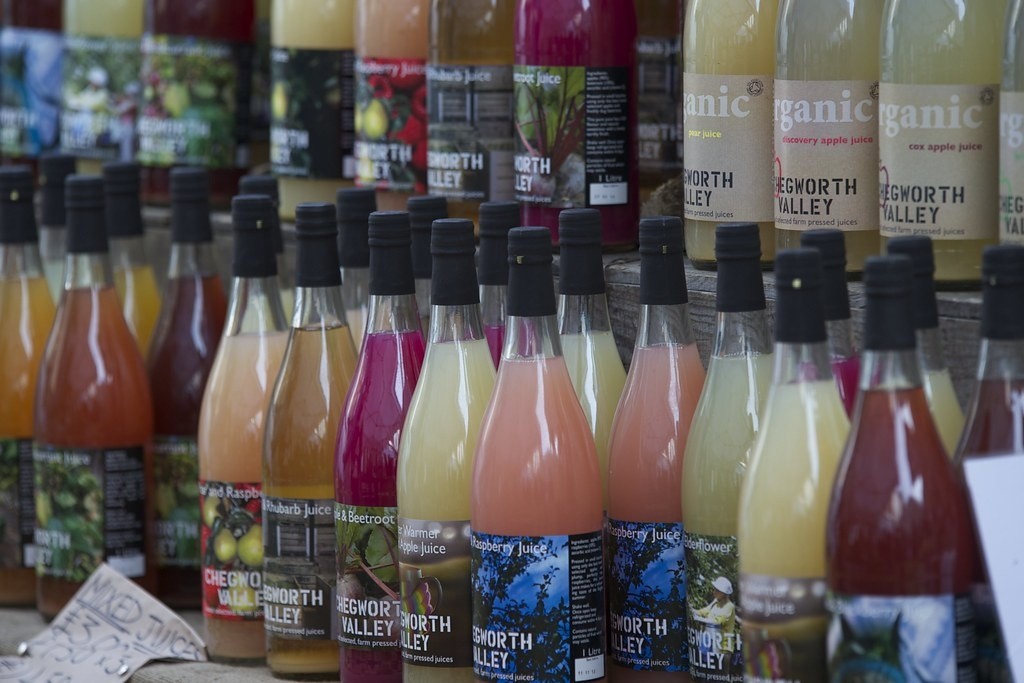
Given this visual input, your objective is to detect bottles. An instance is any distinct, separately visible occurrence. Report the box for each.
[0,0,1024,683]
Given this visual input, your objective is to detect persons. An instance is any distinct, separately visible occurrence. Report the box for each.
[689,576,736,674]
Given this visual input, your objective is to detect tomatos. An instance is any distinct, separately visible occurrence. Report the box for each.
[347,99,388,194]
[201,491,265,613]
